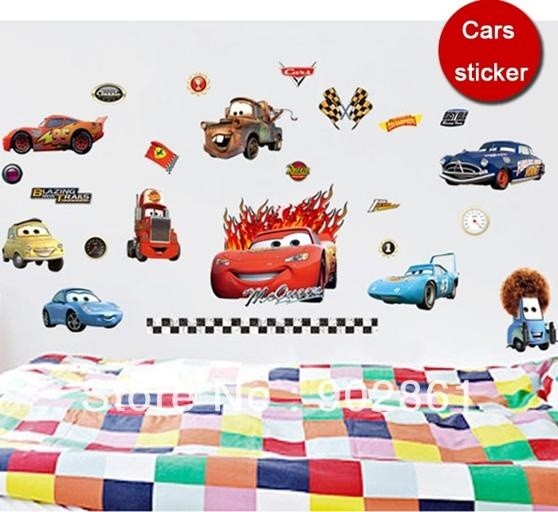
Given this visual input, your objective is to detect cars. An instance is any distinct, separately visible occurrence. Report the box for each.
[368,253,460,309]
[210,227,338,303]
[198,95,283,160]
[439,139,545,189]
[43,287,123,333]
[2,217,64,273]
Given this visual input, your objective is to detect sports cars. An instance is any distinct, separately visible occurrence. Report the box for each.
[2,115,106,154]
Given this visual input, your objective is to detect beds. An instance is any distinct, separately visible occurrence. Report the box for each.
[0,352,558,512]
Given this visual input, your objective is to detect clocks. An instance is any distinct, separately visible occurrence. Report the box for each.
[460,207,489,236]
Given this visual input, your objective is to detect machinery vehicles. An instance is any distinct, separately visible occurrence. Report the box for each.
[127,187,180,261]
